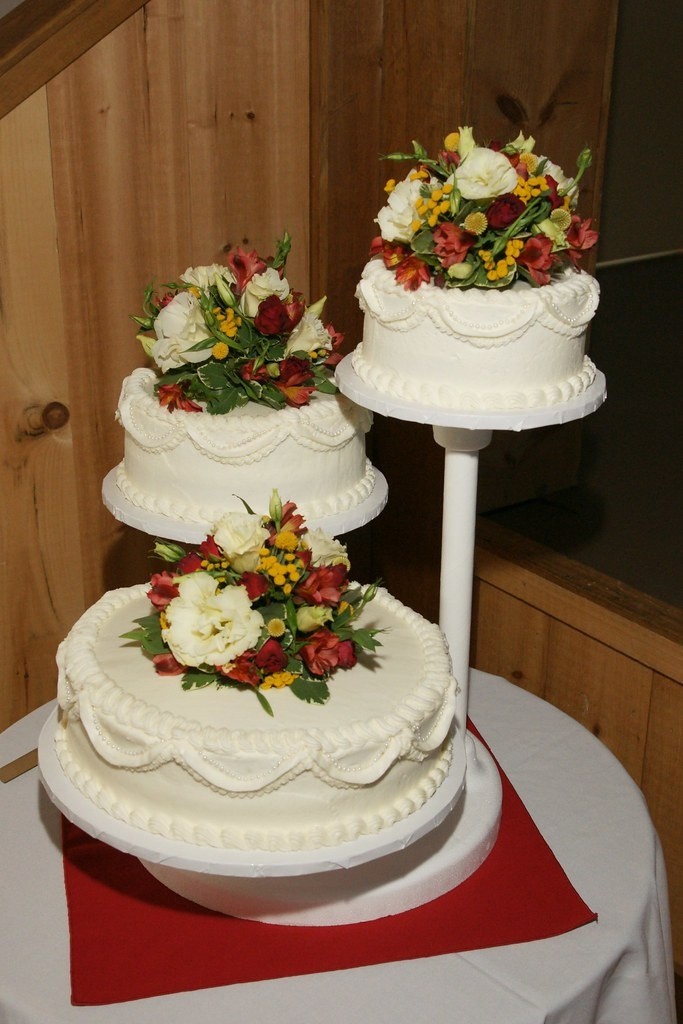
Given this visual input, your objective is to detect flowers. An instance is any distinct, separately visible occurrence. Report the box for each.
[128,230,344,416]
[365,125,597,290]
[117,485,389,716]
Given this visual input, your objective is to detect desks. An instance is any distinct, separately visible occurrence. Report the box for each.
[0,657,677,1024]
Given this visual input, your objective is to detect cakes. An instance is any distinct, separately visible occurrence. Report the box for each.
[116,230,376,525]
[351,126,601,413]
[55,493,460,848]
